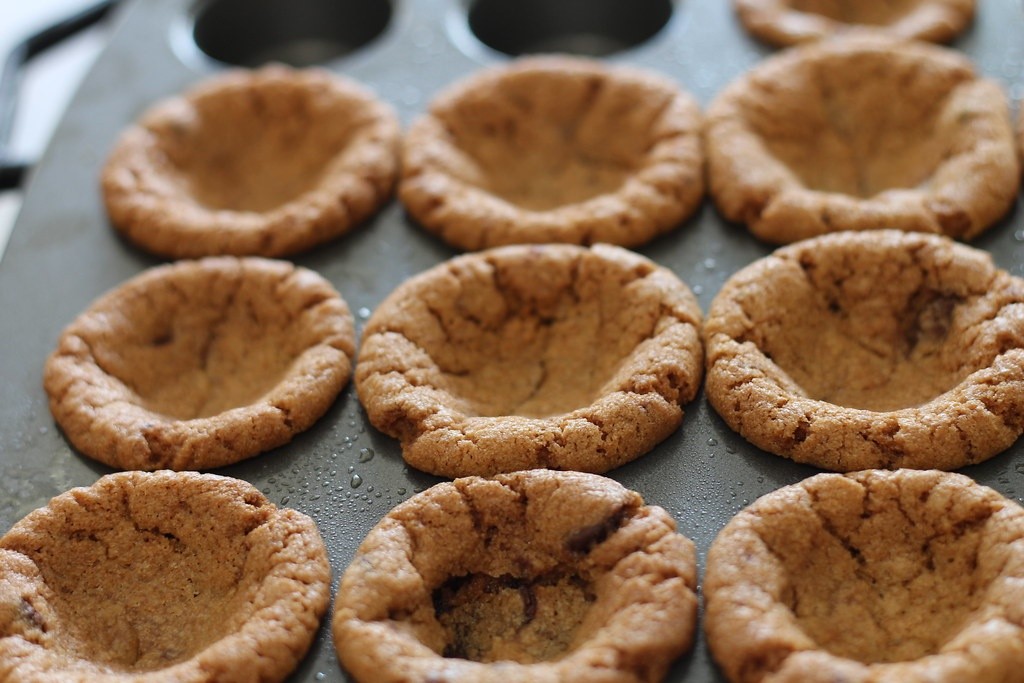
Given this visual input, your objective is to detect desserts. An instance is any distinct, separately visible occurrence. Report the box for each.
[0,0,1023,683]
